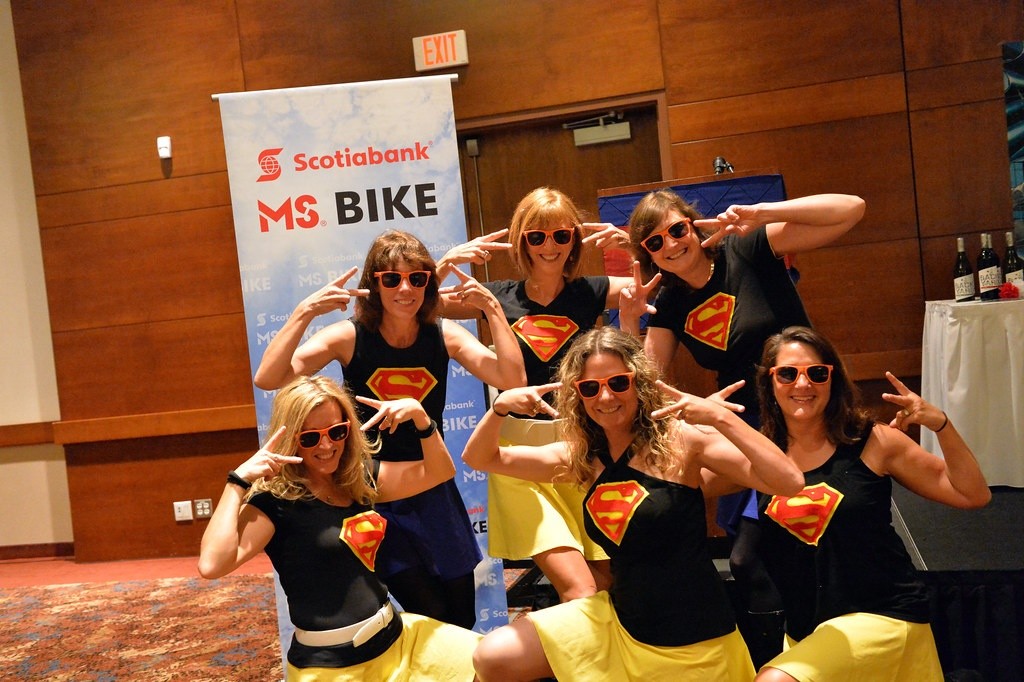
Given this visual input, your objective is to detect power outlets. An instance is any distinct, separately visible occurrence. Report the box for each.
[194,499,213,518]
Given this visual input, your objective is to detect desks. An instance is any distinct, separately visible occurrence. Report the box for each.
[920,297,1024,488]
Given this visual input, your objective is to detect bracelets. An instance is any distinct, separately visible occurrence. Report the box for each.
[414,419,437,439]
[935,411,948,432]
[492,395,510,417]
[225,470,252,489]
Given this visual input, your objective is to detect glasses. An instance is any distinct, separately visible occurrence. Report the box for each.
[770,364,833,385]
[296,418,351,449]
[375,270,431,289]
[640,217,691,253]
[573,371,635,399]
[521,226,576,247]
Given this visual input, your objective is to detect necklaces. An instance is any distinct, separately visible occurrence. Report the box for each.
[706,258,715,282]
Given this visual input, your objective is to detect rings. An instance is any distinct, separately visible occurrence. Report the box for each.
[484,250,489,258]
[534,398,542,406]
[460,290,466,299]
[385,418,392,426]
[901,408,911,417]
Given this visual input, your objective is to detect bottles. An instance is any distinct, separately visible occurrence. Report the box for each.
[952,237,976,303]
[987,235,1004,299]
[1002,232,1024,298]
[977,234,1001,302]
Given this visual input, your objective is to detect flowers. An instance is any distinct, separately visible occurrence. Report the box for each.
[996,278,1024,300]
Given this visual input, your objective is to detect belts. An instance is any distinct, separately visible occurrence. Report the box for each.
[296,602,394,646]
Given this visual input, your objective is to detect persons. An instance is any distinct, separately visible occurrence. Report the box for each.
[692,325,992,682]
[255,224,527,629]
[196,376,491,682]
[434,186,650,607]
[618,188,867,672]
[461,326,804,681]
